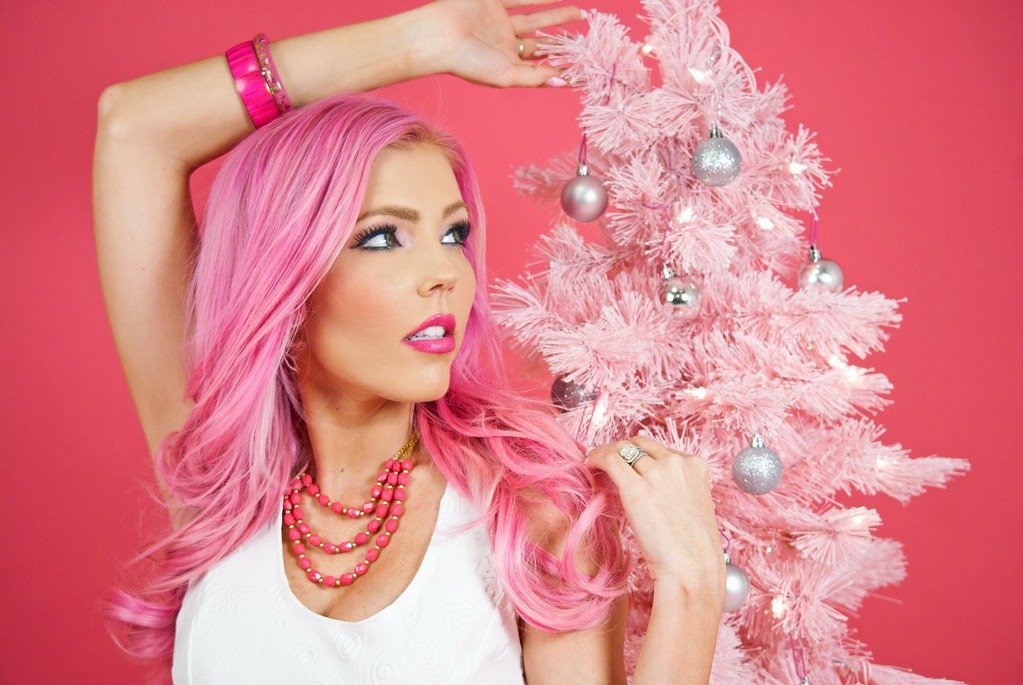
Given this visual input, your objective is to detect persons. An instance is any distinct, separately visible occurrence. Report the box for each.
[92,0,727,685]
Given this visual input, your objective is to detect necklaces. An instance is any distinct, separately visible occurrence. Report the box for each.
[284,432,421,586]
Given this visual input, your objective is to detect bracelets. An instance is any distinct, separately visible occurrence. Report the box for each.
[253,34,293,113]
[226,39,280,129]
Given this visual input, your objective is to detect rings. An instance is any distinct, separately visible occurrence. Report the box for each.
[519,38,524,55]
[620,444,647,468]
